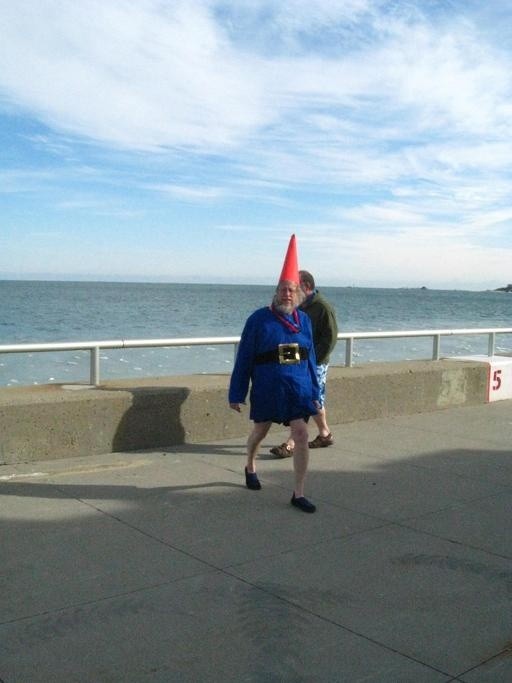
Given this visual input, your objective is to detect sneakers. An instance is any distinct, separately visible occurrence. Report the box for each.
[291,491,316,513]
[245,467,261,490]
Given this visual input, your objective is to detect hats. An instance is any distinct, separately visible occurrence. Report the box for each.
[280,233,300,286]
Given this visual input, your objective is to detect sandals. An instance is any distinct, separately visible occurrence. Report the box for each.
[270,443,294,458]
[307,432,334,448]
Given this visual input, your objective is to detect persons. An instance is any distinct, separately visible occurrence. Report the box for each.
[270,270,339,458]
[228,279,317,514]
